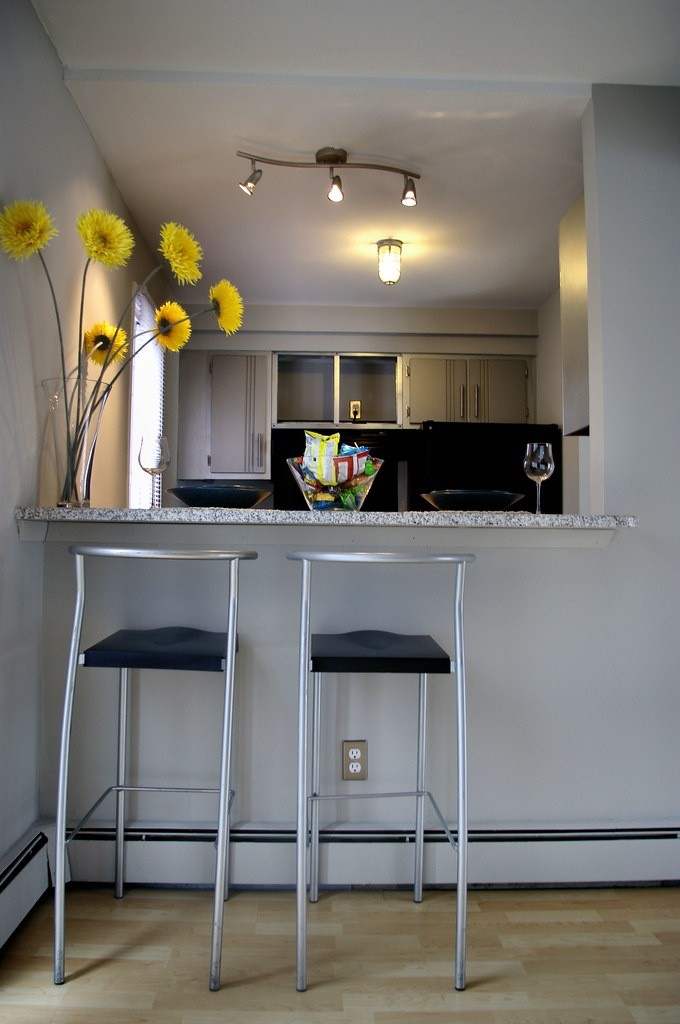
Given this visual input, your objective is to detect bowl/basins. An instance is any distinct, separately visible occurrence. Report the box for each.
[285,457,385,511]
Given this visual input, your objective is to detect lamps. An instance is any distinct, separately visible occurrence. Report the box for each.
[376,238,404,286]
[234,146,422,207]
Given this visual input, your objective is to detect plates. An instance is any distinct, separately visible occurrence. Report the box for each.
[166,487,272,507]
[421,490,524,511]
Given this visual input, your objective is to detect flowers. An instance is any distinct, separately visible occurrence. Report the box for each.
[0,194,246,510]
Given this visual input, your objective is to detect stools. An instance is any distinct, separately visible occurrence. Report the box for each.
[284,547,478,994]
[51,543,261,995]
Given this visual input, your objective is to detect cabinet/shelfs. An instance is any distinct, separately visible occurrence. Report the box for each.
[176,328,538,481]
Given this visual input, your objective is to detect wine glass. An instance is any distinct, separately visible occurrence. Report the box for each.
[524,443,555,514]
[138,435,170,508]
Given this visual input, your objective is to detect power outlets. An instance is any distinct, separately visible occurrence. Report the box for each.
[341,741,368,782]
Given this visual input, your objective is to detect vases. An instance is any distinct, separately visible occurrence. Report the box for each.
[43,377,113,508]
